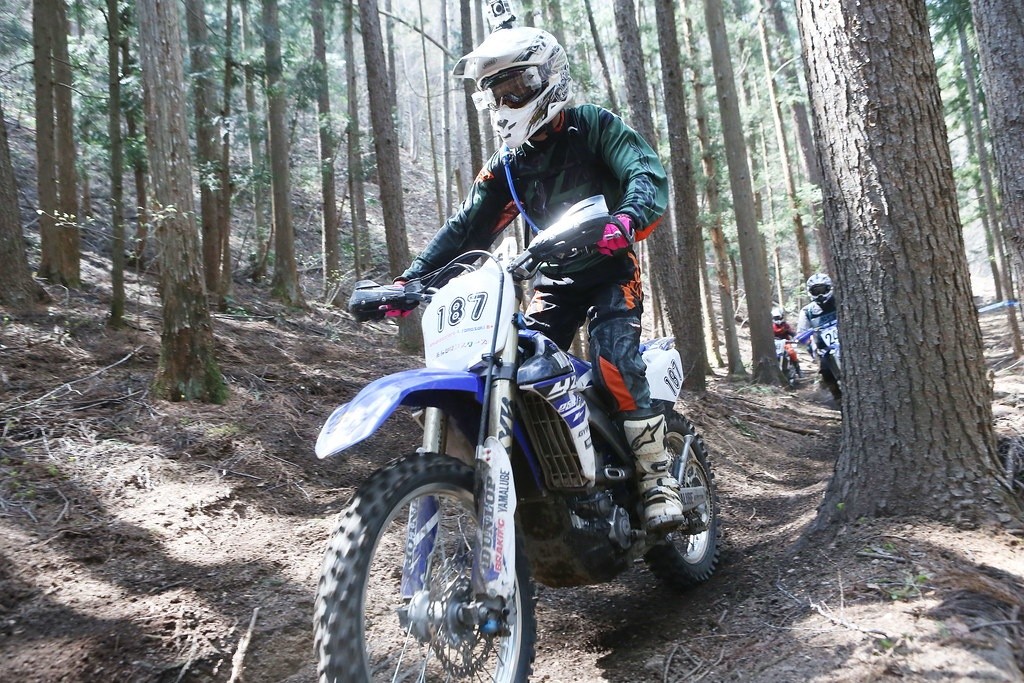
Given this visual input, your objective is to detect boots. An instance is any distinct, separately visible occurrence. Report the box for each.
[623,414,684,531]
[818,369,842,399]
[794,362,804,378]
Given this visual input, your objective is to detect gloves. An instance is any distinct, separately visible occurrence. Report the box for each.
[383,280,419,317]
[596,213,633,255]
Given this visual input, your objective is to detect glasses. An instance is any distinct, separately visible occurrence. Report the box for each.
[808,284,831,296]
[482,69,539,110]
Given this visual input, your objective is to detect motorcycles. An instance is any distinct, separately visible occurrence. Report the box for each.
[773,336,796,380]
[793,311,844,396]
[312,194,721,682]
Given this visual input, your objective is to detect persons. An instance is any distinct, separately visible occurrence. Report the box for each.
[771,306,804,378]
[380,27,683,536]
[795,274,839,360]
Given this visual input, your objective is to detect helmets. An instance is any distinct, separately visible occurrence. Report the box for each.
[771,309,784,326]
[806,273,834,303]
[451,25,574,149]
